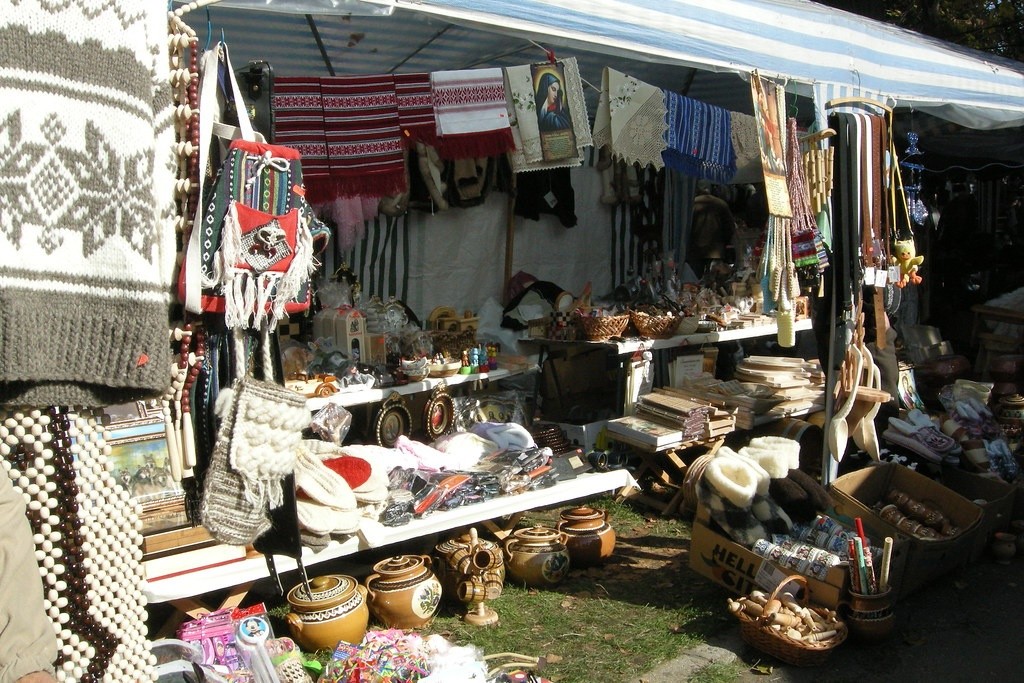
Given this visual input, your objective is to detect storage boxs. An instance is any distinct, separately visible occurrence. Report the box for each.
[917,463,1017,562]
[831,463,985,601]
[688,488,912,613]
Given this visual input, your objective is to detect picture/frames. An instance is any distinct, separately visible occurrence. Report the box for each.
[98,416,186,513]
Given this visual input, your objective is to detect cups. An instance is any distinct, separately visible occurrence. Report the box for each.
[457,577,489,602]
[445,543,474,575]
[471,541,503,570]
[482,571,503,600]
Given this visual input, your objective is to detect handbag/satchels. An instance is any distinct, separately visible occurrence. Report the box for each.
[200,378,311,545]
[178,140,330,333]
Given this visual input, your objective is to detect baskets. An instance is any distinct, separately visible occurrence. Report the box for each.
[630,310,700,340]
[735,575,848,667]
[581,312,630,340]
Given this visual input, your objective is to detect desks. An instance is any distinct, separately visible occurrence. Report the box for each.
[139,318,825,621]
